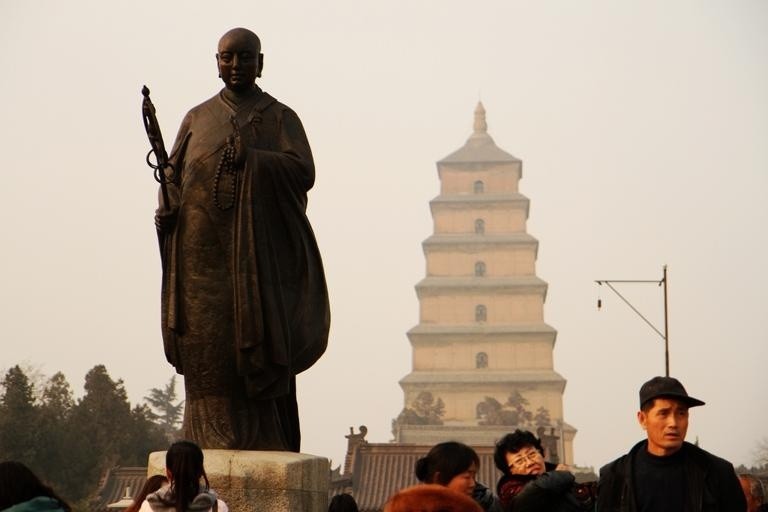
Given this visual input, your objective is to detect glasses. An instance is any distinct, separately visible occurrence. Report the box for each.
[509,449,540,469]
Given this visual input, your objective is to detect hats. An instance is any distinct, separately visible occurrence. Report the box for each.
[642,376,705,409]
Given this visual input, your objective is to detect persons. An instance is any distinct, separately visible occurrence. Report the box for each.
[328,493,358,512]
[492,427,600,512]
[0,460,72,511]
[382,441,485,511]
[139,439,230,511]
[150,25,330,454]
[736,472,768,511]
[123,474,168,512]
[471,479,500,511]
[594,376,748,511]
